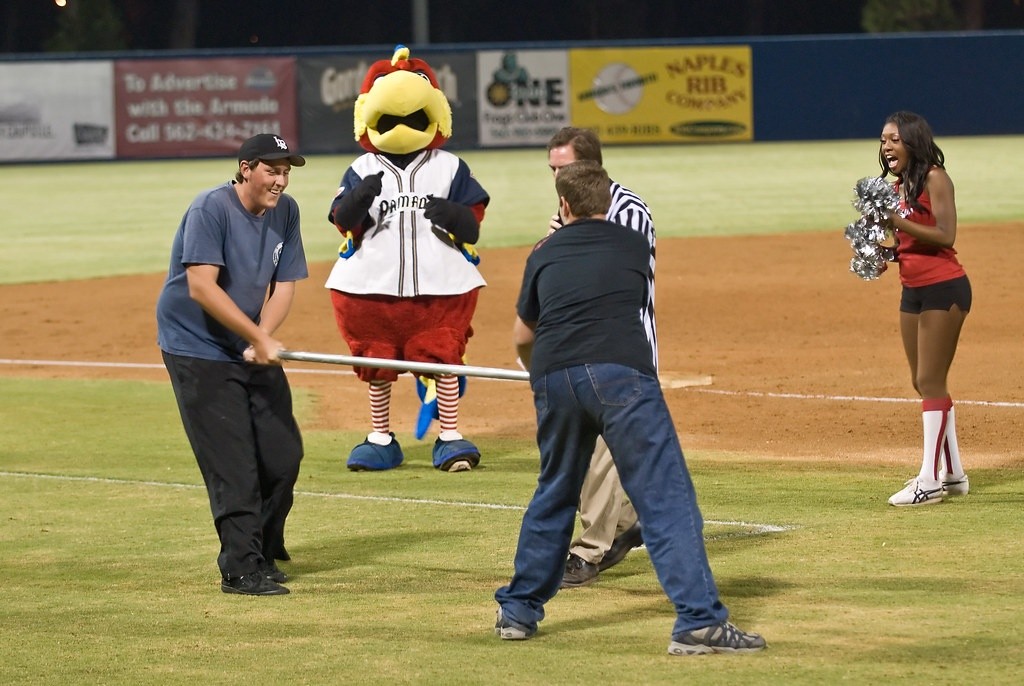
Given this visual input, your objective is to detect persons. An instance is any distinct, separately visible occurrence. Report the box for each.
[851,111,972,508]
[548,127,656,585]
[156,134,308,597]
[495,160,766,656]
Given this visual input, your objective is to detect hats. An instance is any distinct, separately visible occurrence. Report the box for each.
[238,133,306,167]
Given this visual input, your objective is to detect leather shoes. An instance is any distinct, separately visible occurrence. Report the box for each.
[557,553,600,588]
[599,520,644,572]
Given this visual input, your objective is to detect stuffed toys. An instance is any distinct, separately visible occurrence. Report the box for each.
[324,46,490,470]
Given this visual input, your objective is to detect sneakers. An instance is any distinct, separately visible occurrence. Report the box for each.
[938,469,970,495]
[668,621,767,657]
[495,605,530,640]
[260,559,287,583]
[220,574,291,595]
[886,476,944,506]
